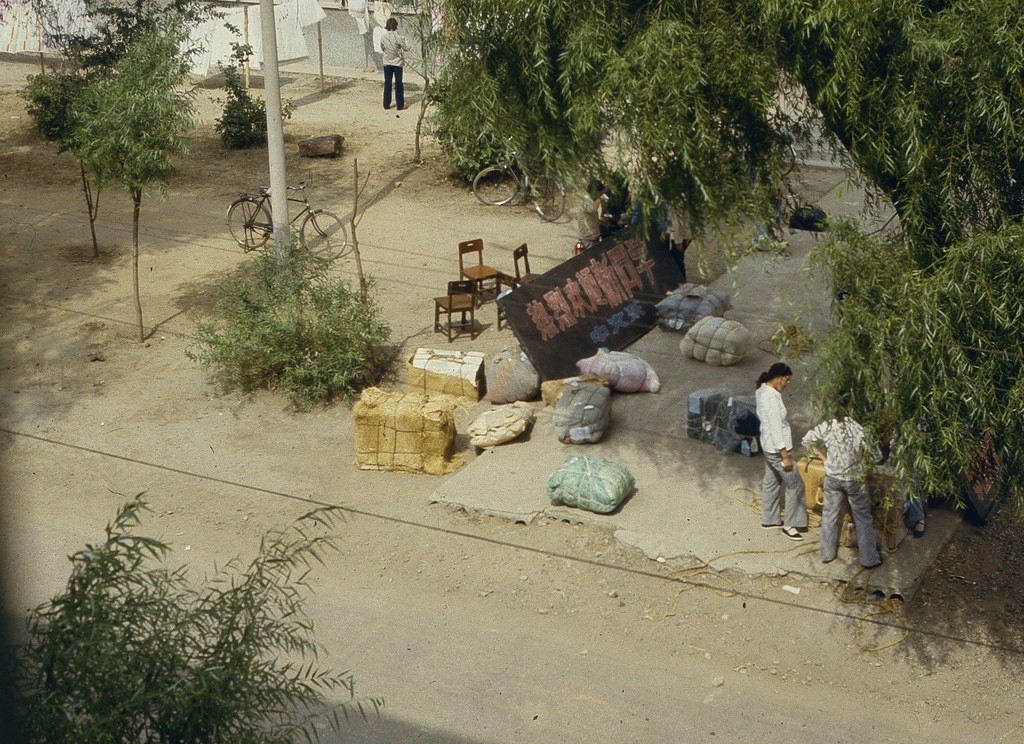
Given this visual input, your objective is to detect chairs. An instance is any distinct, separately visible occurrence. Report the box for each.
[458,239,501,302]
[497,243,543,330]
[434,279,477,342]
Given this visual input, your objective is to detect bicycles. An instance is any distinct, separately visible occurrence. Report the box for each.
[472,136,567,224]
[225,179,349,263]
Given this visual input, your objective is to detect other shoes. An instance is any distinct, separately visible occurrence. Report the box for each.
[782,527,804,541]
[762,521,784,528]
[865,557,883,570]
[913,521,924,538]
[398,106,408,110]
[385,107,391,109]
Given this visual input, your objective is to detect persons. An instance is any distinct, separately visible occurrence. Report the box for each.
[801,390,886,569]
[577,179,692,286]
[754,363,808,541]
[903,486,927,538]
[380,18,411,111]
